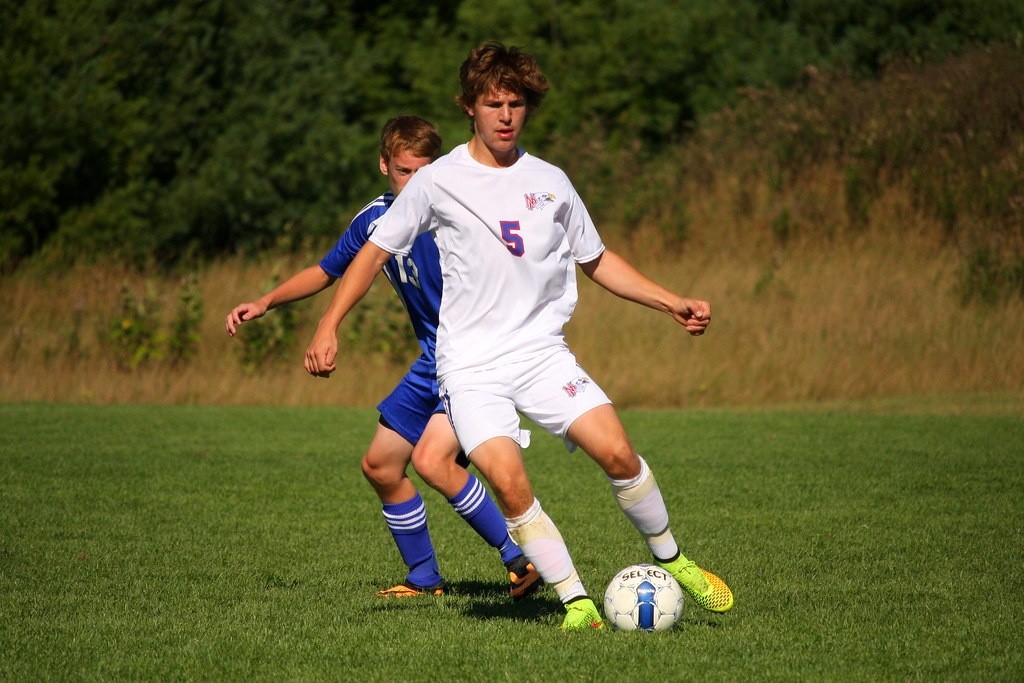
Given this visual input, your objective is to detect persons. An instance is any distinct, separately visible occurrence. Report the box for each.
[304,41,734,630]
[224,116,543,599]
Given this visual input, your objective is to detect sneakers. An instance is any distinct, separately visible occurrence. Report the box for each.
[376,585,443,599]
[653,552,734,614]
[503,553,543,600]
[560,597,607,634]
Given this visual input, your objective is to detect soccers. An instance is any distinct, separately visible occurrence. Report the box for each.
[603,562,686,636]
